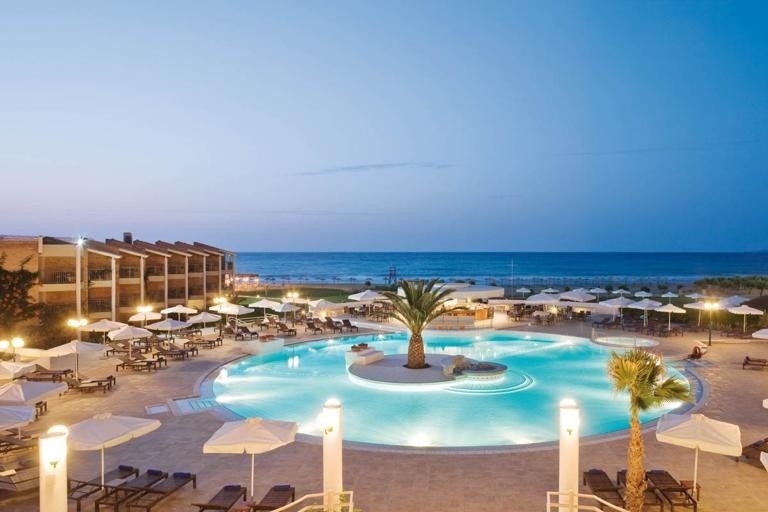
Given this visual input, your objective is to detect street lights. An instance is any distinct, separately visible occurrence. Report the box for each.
[702,301,720,348]
[137,304,153,332]
[1,338,25,381]
[319,397,343,511]
[555,395,581,512]
[287,292,300,327]
[32,424,69,512]
[68,318,89,341]
[213,296,226,338]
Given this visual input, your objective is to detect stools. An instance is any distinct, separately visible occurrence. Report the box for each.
[678,478,702,499]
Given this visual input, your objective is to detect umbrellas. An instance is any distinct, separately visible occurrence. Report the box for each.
[54,337,111,374]
[751,328,768,340]
[67,410,162,489]
[0,405,38,432]
[309,296,338,319]
[0,379,67,439]
[0,359,38,379]
[515,286,765,335]
[348,289,384,314]
[202,416,298,499]
[82,299,302,357]
[656,412,742,495]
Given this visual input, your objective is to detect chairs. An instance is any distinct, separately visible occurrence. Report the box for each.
[614,466,665,512]
[733,439,767,465]
[504,300,766,371]
[578,467,625,512]
[645,469,697,512]
